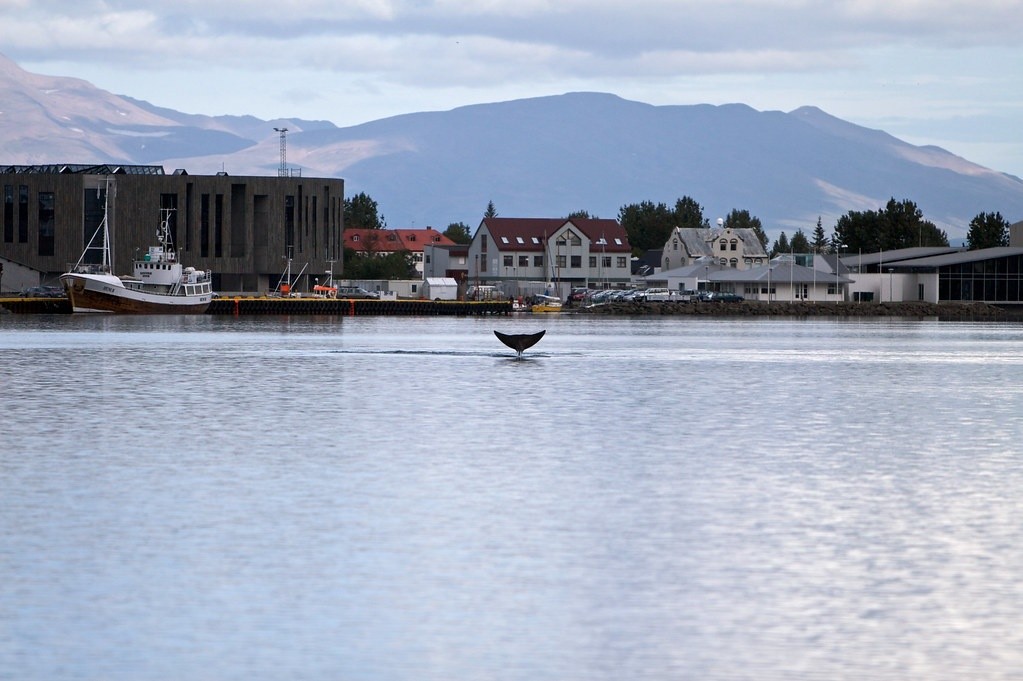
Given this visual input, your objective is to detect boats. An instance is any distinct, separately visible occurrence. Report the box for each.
[59,177,212,315]
[525,259,528,296]
[532,294,563,312]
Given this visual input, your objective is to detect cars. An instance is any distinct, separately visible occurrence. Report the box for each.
[212,292,219,299]
[567,287,745,304]
[27,286,67,298]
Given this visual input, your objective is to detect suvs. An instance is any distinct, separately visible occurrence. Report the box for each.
[467,286,503,300]
[336,286,379,299]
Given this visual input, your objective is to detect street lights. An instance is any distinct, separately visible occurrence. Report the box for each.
[835,245,848,304]
[288,245,294,288]
[768,267,773,300]
